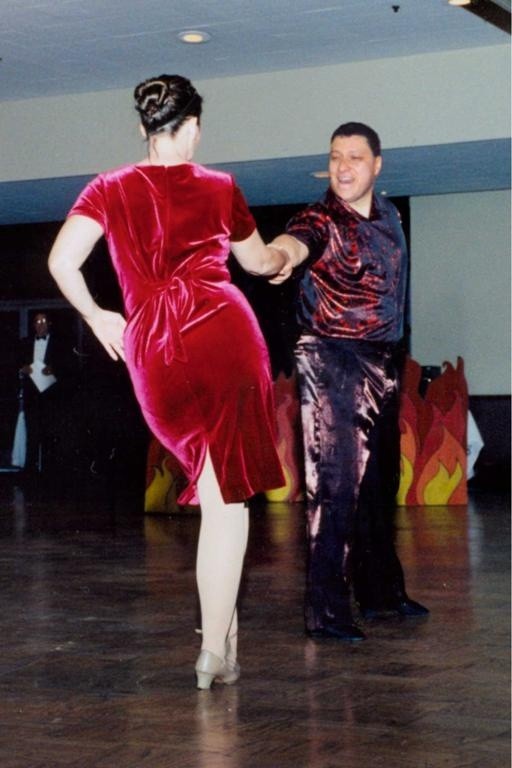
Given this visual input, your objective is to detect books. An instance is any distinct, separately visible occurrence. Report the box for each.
[29,360,57,393]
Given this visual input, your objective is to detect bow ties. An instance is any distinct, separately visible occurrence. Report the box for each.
[36,335,47,341]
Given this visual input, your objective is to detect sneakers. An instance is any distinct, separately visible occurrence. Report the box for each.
[392,598,428,618]
[320,622,367,641]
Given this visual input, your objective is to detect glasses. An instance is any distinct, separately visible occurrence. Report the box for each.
[35,319,46,323]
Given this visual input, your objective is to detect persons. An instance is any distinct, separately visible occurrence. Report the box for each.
[48,75,290,690]
[17,312,75,473]
[265,122,429,640]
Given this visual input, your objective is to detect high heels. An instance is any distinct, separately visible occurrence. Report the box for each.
[194,650,240,691]
